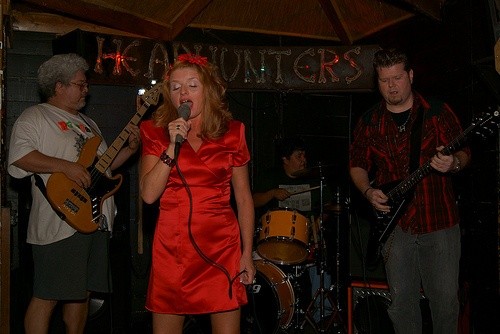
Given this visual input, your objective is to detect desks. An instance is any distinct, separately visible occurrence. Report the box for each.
[348,280,470,334]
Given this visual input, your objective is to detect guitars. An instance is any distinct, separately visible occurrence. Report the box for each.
[355,107,500,244]
[45,80,165,234]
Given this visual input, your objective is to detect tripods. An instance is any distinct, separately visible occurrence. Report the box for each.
[299,168,348,334]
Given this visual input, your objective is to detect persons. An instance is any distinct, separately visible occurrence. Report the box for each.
[6,53,139,334]
[250,139,332,322]
[138,53,255,334]
[348,48,471,334]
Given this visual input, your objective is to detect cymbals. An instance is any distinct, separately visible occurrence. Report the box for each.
[291,165,340,176]
[322,205,347,213]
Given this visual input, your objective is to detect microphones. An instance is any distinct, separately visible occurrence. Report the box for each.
[174,104,191,155]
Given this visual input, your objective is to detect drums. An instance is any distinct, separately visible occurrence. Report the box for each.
[240,259,295,334]
[255,207,312,265]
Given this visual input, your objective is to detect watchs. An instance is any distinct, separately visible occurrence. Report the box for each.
[452,155,464,173]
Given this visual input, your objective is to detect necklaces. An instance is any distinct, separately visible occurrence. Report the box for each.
[391,106,412,133]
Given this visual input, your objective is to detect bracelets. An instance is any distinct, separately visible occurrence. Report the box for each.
[159,151,176,168]
[362,187,372,196]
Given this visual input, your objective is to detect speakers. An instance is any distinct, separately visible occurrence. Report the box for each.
[348,282,434,334]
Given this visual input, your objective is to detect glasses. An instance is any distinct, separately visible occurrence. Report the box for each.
[68,81,88,92]
